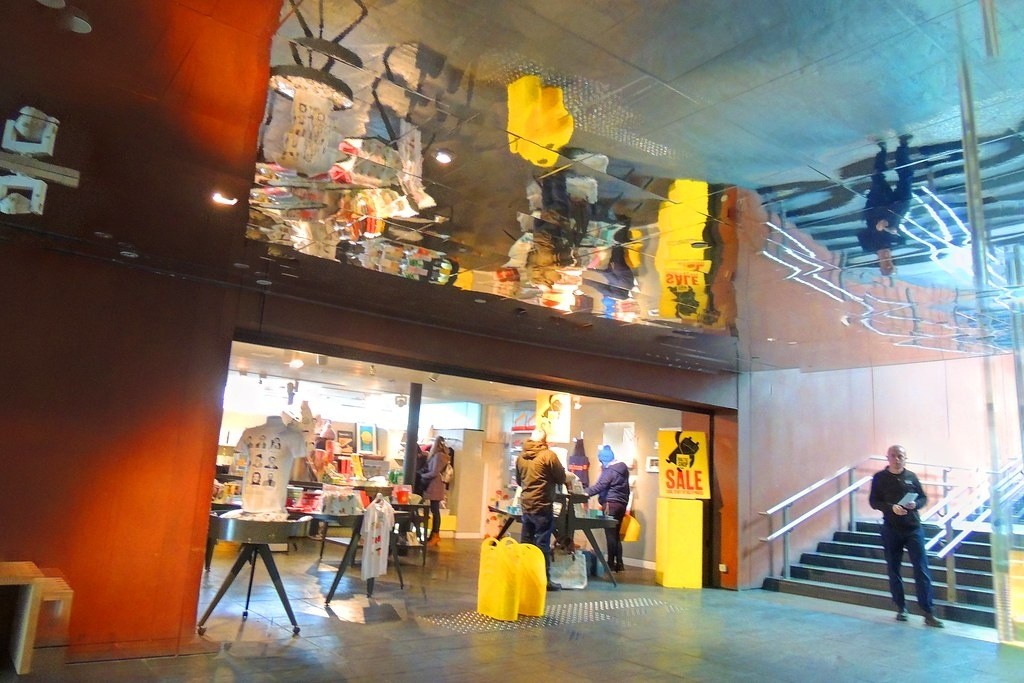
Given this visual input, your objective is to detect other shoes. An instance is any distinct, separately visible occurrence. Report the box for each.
[607,563,616,573]
[897,134,912,144]
[896,608,909,621]
[877,141,887,151]
[614,563,625,572]
[925,613,944,627]
[547,581,560,591]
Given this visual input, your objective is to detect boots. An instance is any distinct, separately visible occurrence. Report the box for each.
[426,532,440,547]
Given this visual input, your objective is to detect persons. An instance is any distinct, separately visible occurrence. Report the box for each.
[585,445,630,575]
[869,445,948,629]
[516,428,567,591]
[857,133,914,278]
[527,167,634,312]
[414,435,451,548]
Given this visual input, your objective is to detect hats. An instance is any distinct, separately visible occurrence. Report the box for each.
[598,445,614,471]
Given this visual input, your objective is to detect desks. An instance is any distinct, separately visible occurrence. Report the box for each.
[303,510,413,606]
[488,492,622,587]
[198,508,315,633]
[319,494,435,565]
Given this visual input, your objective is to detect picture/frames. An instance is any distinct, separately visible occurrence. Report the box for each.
[356,422,378,454]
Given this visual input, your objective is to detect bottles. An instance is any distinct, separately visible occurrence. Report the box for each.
[388,469,403,484]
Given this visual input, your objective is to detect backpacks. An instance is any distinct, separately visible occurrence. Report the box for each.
[440,461,453,483]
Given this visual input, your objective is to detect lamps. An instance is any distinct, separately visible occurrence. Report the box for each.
[395,395,407,407]
